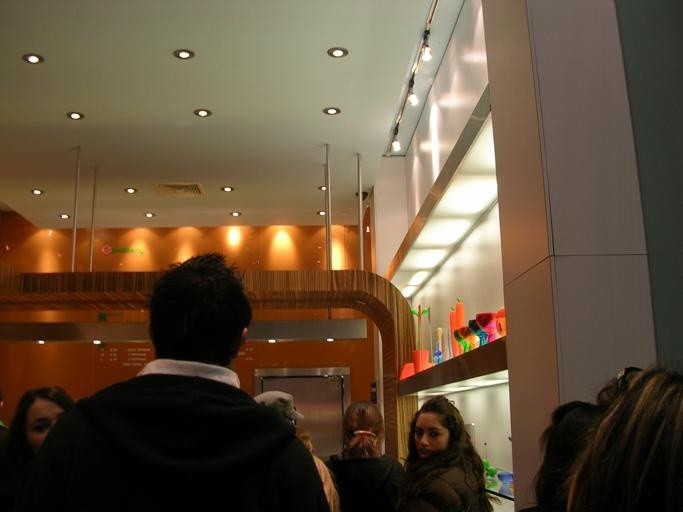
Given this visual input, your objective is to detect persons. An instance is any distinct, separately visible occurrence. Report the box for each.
[1,386,75,487]
[396,395,493,512]
[325,401,407,511]
[530,365,683,512]
[255,391,340,512]
[19,253,331,512]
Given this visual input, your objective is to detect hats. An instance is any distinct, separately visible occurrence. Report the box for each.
[252,390,306,421]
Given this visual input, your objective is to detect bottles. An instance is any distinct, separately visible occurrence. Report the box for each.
[433,351,444,366]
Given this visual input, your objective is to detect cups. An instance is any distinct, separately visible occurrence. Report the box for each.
[411,350,430,375]
[453,312,498,353]
